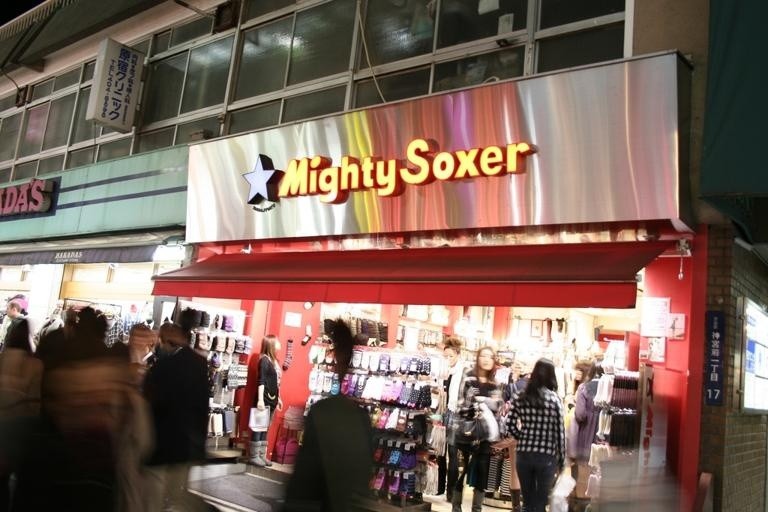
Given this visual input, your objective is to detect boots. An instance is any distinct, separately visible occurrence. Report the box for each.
[508,488,522,512]
[248,441,273,468]
[471,489,485,512]
[451,490,462,512]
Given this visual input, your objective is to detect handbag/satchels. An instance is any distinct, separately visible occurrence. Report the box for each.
[487,455,513,498]
[453,418,487,452]
